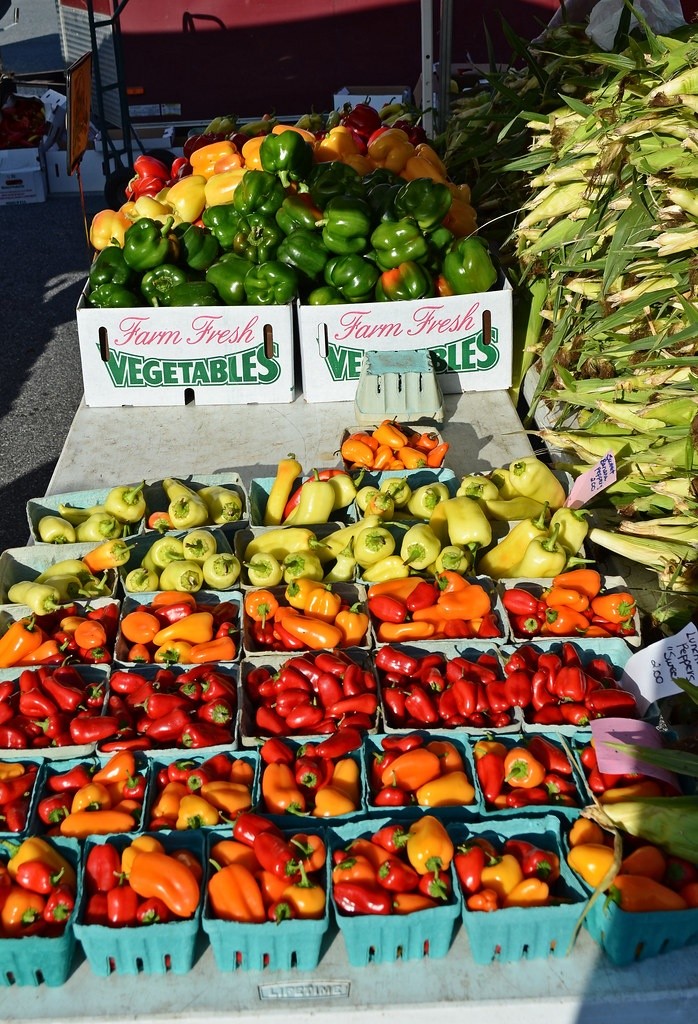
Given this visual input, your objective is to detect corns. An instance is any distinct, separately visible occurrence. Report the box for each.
[444,33,698,865]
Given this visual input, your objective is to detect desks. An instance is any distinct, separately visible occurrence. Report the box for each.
[0,391,698,1024]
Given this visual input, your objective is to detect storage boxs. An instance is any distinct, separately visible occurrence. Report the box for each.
[0,96,47,205]
[413,64,509,109]
[75,273,298,404]
[340,426,447,471]
[353,349,445,431]
[46,123,185,193]
[333,85,411,112]
[0,467,698,986]
[298,267,513,404]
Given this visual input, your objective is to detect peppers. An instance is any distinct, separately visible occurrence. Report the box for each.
[86,95,497,308]
[0,97,47,150]
[0,452,698,953]
[338,414,449,470]
